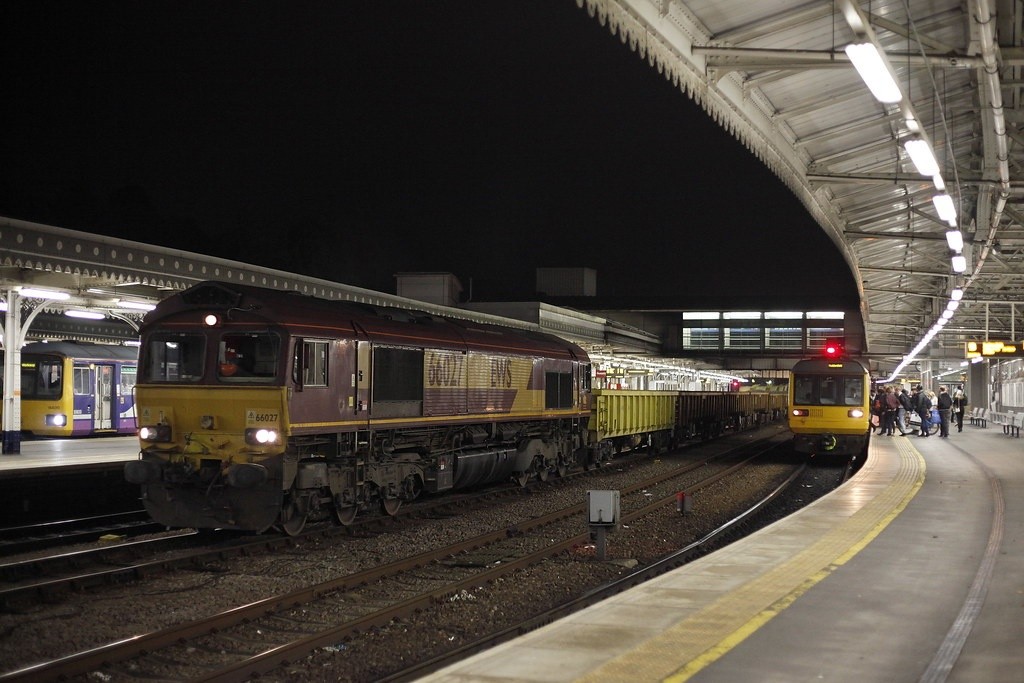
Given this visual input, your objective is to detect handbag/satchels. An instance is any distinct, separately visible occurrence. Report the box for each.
[921,419,929,431]
[875,399,880,410]
[953,407,960,413]
[923,396,932,409]
[872,415,880,426]
[931,410,941,423]
[910,414,922,423]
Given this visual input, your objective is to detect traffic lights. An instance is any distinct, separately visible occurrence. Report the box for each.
[733,382,738,391]
[826,347,835,355]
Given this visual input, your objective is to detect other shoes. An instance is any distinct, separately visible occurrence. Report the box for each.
[877,431,886,435]
[918,434,924,436]
[887,433,891,435]
[873,426,877,432]
[899,433,906,436]
[924,434,931,438]
[938,435,943,437]
[958,428,962,431]
[944,434,948,437]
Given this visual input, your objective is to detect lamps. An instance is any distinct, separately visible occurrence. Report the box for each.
[932,194,957,221]
[945,230,963,250]
[887,288,963,382]
[18,289,71,300]
[64,310,105,319]
[844,33,902,104]
[952,256,967,272]
[116,300,156,310]
[904,133,940,175]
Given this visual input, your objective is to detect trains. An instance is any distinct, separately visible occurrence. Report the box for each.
[789,355,870,457]
[132,280,790,538]
[20,340,140,440]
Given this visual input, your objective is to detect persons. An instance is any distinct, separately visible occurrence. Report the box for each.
[871,383,968,439]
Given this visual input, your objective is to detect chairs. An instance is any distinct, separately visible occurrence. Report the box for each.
[967,407,978,425]
[977,409,990,428]
[1008,412,1024,438]
[1001,411,1014,435]
[972,408,985,426]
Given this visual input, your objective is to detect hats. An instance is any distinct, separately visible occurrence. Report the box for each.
[901,389,906,392]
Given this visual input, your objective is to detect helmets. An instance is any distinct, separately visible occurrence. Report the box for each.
[221,365,237,376]
